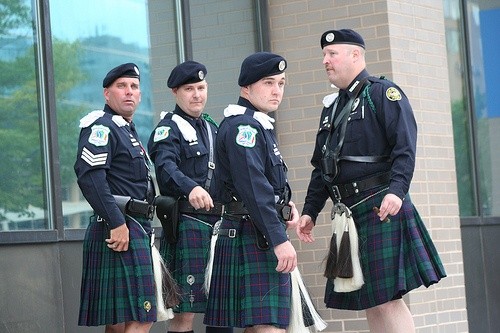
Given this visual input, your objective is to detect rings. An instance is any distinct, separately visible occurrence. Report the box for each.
[112,242,118,247]
[307,233,311,236]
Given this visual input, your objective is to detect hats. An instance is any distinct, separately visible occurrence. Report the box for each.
[321,29,365,49]
[167,61,207,88]
[238,52,287,86]
[103,63,140,87]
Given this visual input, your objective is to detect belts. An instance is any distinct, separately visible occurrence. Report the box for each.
[179,201,222,216]
[227,202,292,221]
[328,170,390,200]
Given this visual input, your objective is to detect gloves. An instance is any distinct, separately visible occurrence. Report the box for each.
[160,112,198,143]
[322,84,340,108]
[79,110,128,128]
[223,104,275,130]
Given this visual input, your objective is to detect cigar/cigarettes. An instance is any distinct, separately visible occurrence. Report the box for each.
[373,206,390,224]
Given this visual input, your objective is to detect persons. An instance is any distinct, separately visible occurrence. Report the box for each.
[295,27,448,333]
[72,62,181,333]
[147,60,225,332]
[202,50,328,333]
